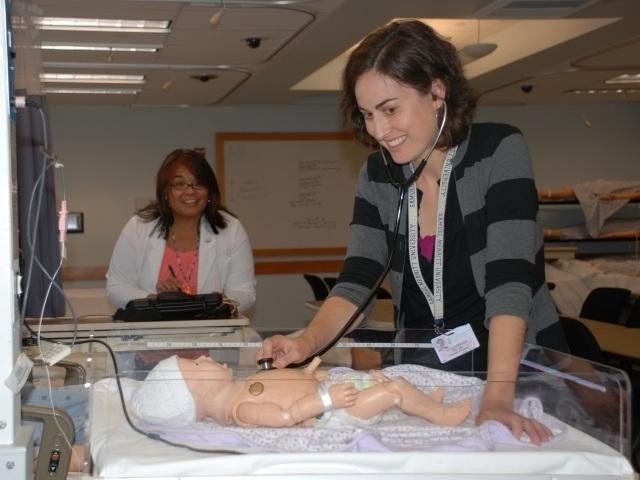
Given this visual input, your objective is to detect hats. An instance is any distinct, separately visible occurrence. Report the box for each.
[128,353,201,429]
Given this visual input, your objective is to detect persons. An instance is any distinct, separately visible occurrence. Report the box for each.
[129,349,474,430]
[252,18,585,448]
[103,147,259,382]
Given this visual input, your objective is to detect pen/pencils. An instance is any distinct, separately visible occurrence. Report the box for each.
[168,265,182,292]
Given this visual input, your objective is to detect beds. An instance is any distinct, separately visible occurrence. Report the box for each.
[79,360,635,480]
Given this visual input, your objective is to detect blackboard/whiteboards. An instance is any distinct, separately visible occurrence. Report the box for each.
[215,131,378,256]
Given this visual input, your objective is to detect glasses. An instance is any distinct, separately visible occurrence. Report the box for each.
[170,179,207,190]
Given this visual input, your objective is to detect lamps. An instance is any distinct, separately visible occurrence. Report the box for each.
[40,14,173,98]
[457,19,498,72]
